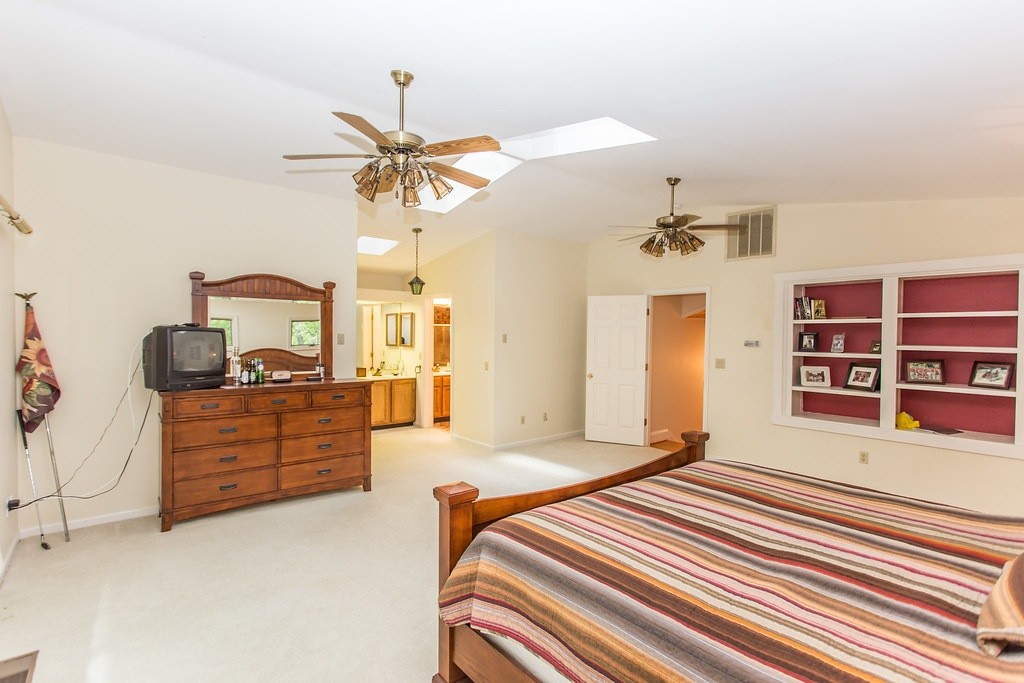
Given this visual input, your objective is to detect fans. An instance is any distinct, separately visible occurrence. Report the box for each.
[608,176,751,242]
[281,69,501,190]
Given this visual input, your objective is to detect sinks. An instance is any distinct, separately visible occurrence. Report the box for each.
[368,376,397,377]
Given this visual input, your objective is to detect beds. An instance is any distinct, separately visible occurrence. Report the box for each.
[430,429,1024,683]
[225,348,320,374]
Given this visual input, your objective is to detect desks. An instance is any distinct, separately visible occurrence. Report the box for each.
[434,374,451,424]
[370,373,416,430]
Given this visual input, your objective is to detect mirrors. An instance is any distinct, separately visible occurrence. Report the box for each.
[386,315,397,346]
[357,302,399,373]
[400,314,412,346]
[187,270,337,379]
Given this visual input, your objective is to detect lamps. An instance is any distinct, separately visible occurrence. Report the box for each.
[352,157,453,209]
[641,230,708,258]
[407,227,426,296]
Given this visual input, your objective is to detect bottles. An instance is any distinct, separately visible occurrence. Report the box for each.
[319,363,325,378]
[230,347,264,384]
[316,363,319,372]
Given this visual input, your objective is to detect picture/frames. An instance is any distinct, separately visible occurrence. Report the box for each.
[967,360,1015,391]
[870,340,882,354]
[829,334,845,353]
[799,365,832,387]
[797,332,819,353]
[841,362,881,392]
[903,358,946,385]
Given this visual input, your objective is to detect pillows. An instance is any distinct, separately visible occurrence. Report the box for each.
[976,551,1024,657]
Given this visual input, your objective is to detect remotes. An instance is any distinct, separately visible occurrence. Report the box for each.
[183,323,200,327]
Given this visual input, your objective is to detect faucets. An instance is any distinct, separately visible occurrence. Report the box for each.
[372,368,383,376]
[434,364,440,372]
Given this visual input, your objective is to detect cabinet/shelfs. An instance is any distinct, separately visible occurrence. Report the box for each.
[432,304,453,369]
[894,267,1020,445]
[157,377,373,532]
[791,277,885,428]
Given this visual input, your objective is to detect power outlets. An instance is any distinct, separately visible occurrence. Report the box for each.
[714,359,726,369]
[858,451,868,465]
[5,496,14,518]
[521,416,526,425]
[543,412,550,423]
[484,361,489,371]
[502,333,508,344]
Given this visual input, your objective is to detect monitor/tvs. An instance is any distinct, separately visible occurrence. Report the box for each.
[142,326,227,392]
